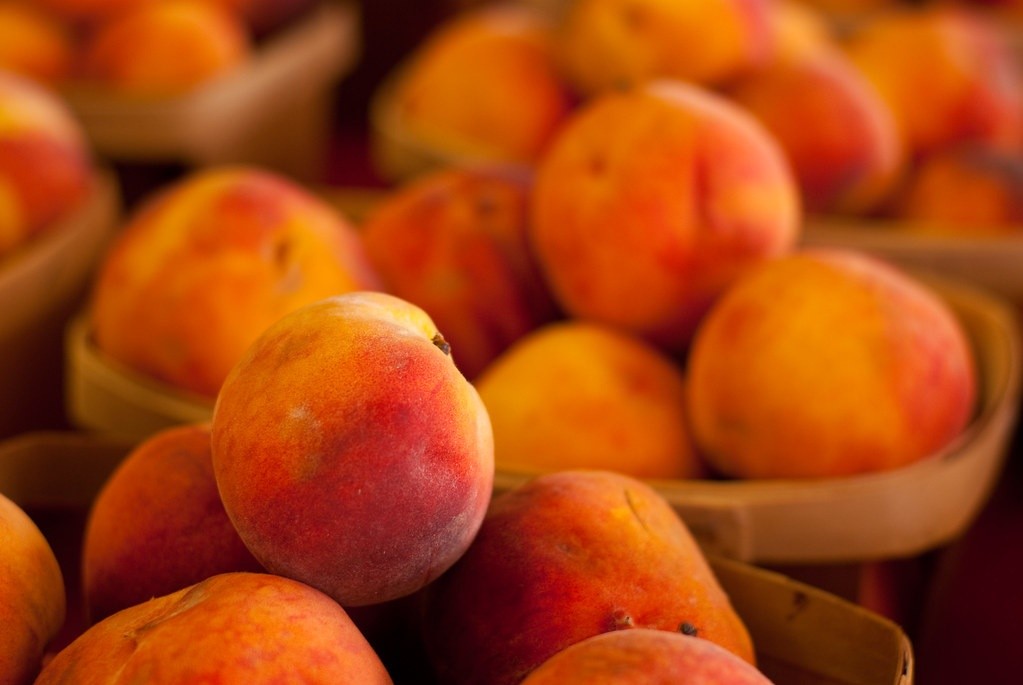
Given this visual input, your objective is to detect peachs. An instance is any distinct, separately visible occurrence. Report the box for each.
[0,0,1023,685]
